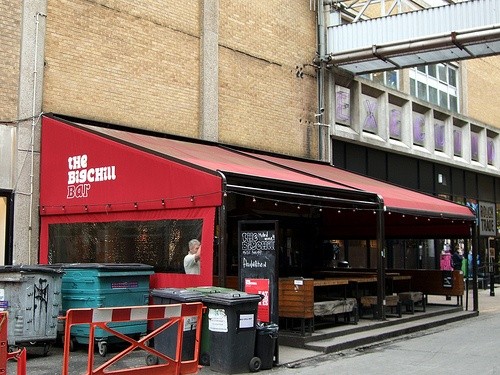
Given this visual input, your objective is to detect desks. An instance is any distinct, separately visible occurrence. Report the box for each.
[311,274,412,319]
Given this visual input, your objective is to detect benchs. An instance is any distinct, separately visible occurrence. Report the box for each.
[398,291,427,315]
[312,297,357,331]
[360,294,403,318]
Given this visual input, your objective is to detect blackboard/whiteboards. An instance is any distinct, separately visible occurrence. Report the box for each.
[238,221,279,362]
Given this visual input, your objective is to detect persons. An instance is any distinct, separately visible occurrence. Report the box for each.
[184,239,200,275]
[441,243,464,300]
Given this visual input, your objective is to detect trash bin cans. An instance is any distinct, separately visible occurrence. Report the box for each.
[39,263,155,356]
[146,288,205,365]
[254,321,279,369]
[202,293,261,374]
[185,287,235,365]
[0,265,66,361]
